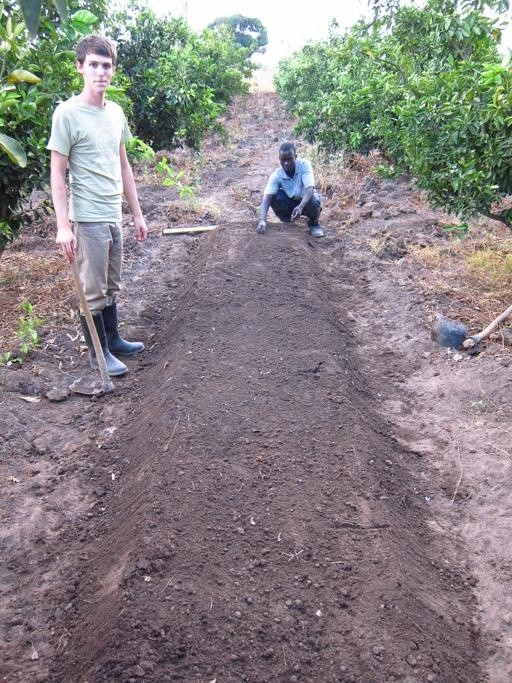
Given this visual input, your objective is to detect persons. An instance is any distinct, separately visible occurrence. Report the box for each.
[45,34,148,377]
[256,143,325,238]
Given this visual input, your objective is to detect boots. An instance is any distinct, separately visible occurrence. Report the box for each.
[103,303,145,355]
[77,309,128,376]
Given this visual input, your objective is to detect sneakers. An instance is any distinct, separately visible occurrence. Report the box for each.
[309,225,324,237]
